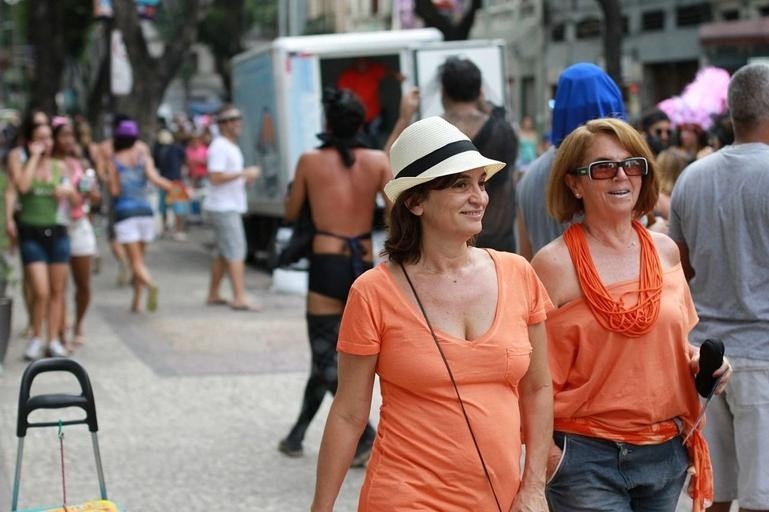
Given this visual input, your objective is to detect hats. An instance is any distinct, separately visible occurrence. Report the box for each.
[382,116,506,205]
[114,120,140,139]
[214,103,243,122]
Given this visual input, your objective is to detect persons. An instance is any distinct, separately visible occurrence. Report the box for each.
[1,100,262,362]
[513,60,769,512]
[276,58,517,469]
[312,117,557,512]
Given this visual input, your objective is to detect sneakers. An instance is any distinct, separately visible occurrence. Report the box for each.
[280,441,303,457]
[25,337,46,361]
[351,426,376,466]
[47,339,66,358]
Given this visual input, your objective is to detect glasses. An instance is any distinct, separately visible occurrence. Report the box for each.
[569,157,649,180]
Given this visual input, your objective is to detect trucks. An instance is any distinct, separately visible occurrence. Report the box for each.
[221,28,511,275]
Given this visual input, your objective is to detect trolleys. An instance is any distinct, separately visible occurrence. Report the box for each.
[10,355,119,512]
